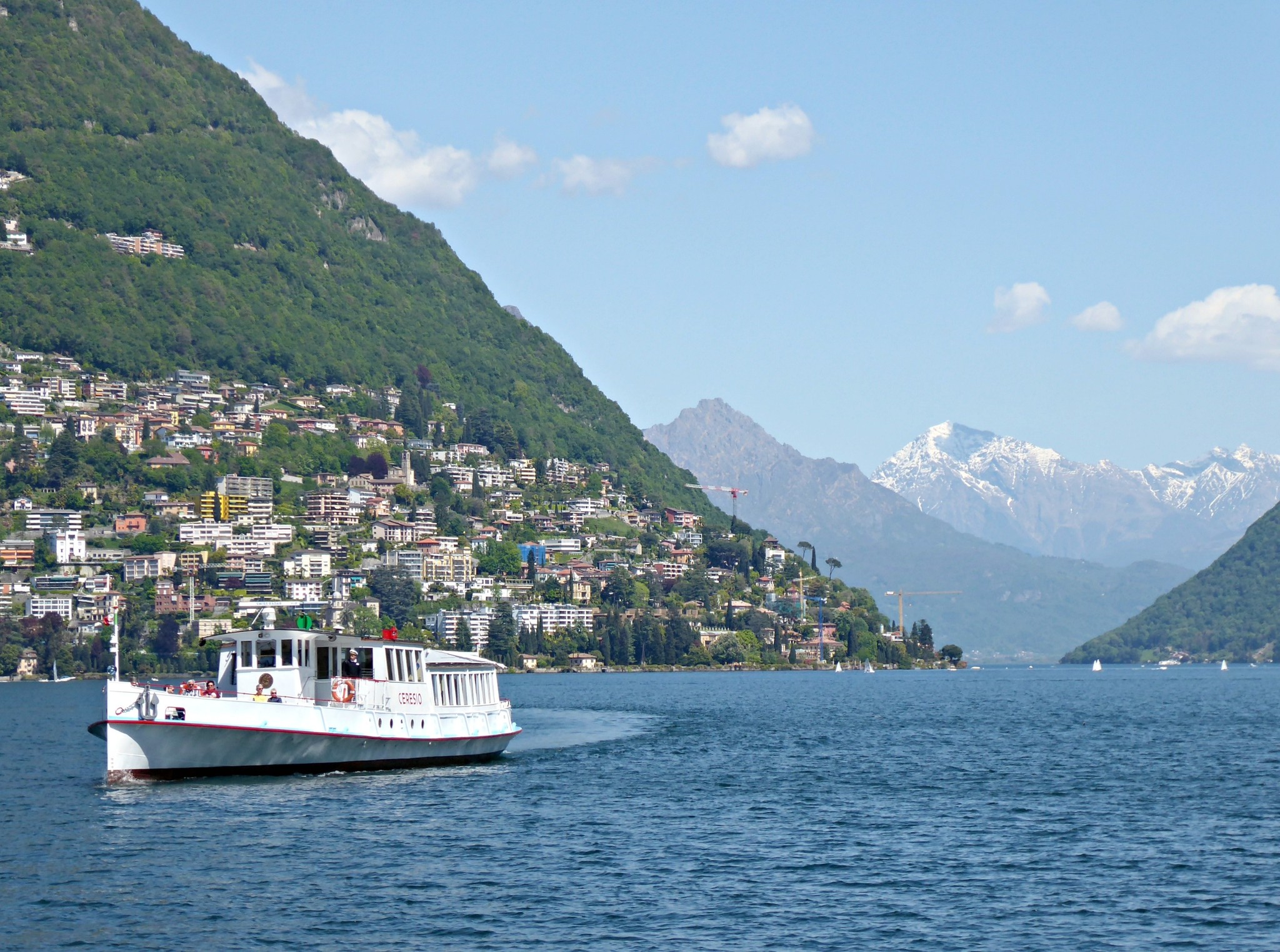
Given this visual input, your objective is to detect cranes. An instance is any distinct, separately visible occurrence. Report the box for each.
[793,568,818,619]
[884,588,964,636]
[685,482,749,534]
[798,594,827,663]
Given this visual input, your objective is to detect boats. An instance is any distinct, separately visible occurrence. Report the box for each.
[1249,663,1257,667]
[950,666,957,671]
[87,595,523,784]
[1159,667,1167,670]
[1159,659,1181,665]
[971,666,981,669]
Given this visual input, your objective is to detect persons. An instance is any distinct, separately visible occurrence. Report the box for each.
[181,679,201,697]
[267,688,282,703]
[342,649,362,678]
[252,684,268,702]
[201,680,220,698]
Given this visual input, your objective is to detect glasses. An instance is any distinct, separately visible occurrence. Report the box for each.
[189,684,194,686]
[257,688,263,690]
[271,692,276,694]
[207,685,213,686]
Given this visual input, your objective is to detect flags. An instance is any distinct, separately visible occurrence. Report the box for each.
[102,613,114,626]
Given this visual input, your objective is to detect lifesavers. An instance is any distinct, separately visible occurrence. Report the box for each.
[331,680,355,702]
[179,684,202,695]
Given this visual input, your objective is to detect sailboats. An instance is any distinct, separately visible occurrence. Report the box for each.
[1092,659,1102,671]
[864,658,875,673]
[1221,659,1229,671]
[835,661,844,673]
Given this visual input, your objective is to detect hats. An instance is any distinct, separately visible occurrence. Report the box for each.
[350,649,358,655]
